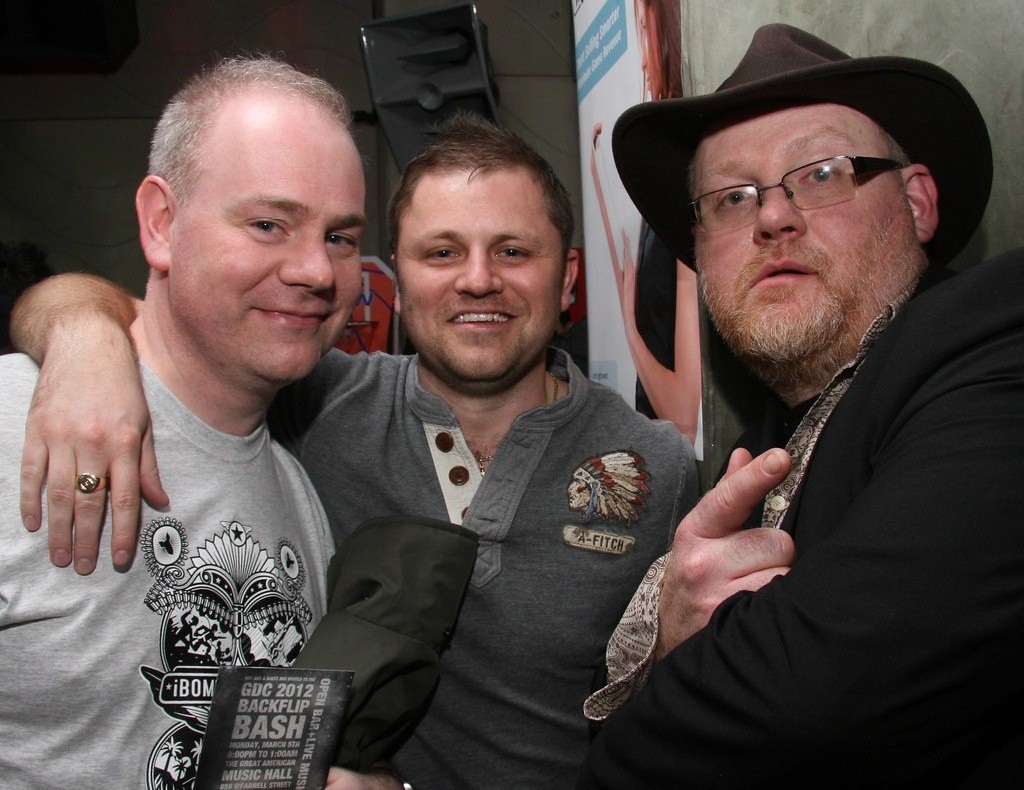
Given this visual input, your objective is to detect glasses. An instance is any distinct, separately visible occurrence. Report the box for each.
[686,155,907,237]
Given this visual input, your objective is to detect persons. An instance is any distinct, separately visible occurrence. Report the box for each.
[10,111,699,790]
[0,59,414,790]
[578,24,1023,790]
[590,0,699,440]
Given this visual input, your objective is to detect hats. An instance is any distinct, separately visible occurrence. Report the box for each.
[609,23,994,273]
[292,515,480,766]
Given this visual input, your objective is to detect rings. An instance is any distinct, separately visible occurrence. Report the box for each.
[75,472,109,493]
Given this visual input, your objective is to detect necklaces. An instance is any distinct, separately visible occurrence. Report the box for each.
[475,370,558,477]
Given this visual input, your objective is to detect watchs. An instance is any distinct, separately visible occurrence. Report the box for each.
[367,767,414,790]
